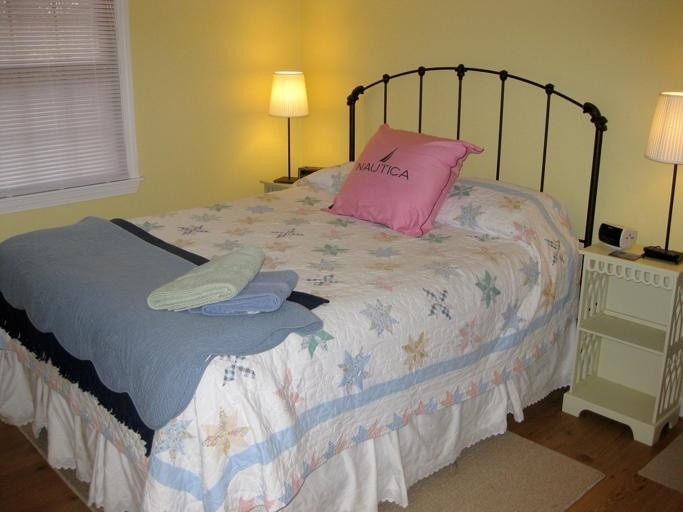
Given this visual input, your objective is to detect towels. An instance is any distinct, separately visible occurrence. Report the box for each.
[0,217,320,431]
[146,247,264,310]
[197,268,298,314]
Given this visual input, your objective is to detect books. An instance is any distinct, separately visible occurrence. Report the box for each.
[608,249,640,263]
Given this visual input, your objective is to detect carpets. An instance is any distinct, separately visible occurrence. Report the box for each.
[374,430,605,512]
[639,436,683,491]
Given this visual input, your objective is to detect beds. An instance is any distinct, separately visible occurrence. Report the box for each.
[0,65,609,512]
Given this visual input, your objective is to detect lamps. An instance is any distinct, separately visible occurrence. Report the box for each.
[268,72,310,184]
[645,91,683,263]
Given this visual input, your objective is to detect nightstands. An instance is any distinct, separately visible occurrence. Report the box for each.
[563,244,682,447]
[260,178,290,195]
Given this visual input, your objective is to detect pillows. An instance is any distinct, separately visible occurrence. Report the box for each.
[323,122,484,239]
